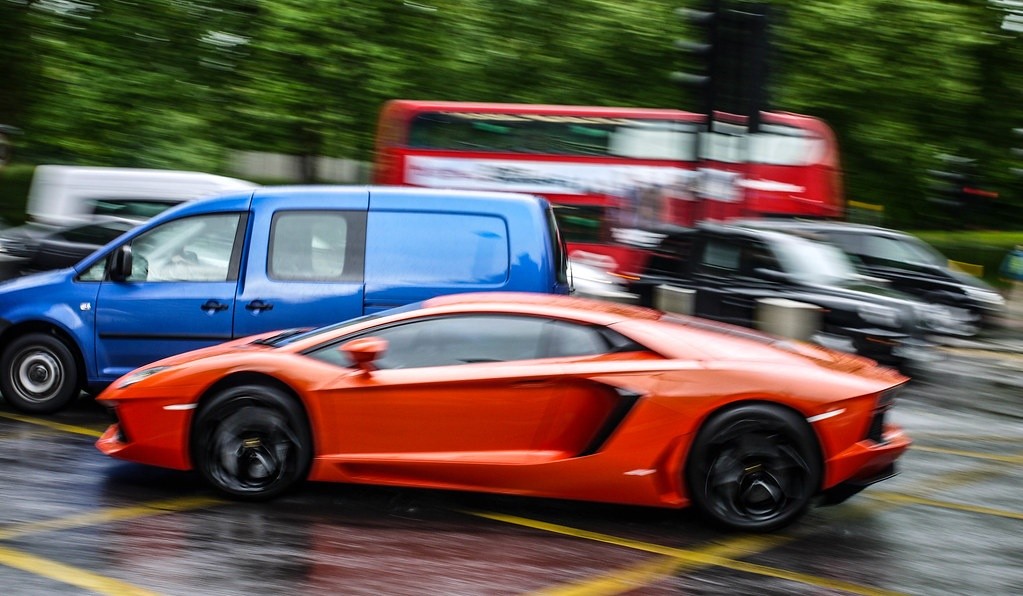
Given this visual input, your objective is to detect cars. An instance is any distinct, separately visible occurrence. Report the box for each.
[629,216,1004,395]
[0,215,153,282]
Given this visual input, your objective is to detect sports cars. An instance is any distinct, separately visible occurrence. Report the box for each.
[95,291,916,537]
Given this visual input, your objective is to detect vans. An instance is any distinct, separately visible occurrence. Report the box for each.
[24,164,333,270]
[0,184,574,420]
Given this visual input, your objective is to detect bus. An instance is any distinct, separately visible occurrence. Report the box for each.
[376,96,845,284]
